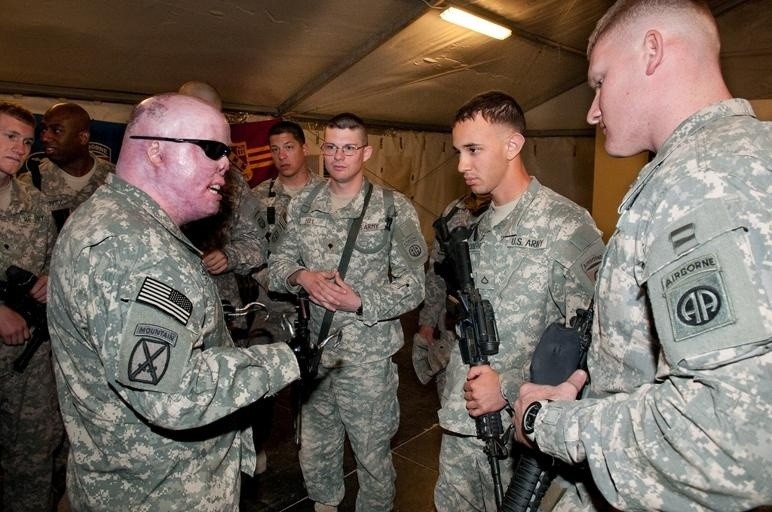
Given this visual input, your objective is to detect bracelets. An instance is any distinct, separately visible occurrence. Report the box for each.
[356,303,363,317]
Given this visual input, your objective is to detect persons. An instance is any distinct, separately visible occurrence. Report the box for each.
[511,2,772,512]
[0,102,64,512]
[14,101,117,512]
[176,79,269,509]
[246,120,329,291]
[411,187,494,409]
[44,90,302,512]
[266,111,430,512]
[438,90,607,512]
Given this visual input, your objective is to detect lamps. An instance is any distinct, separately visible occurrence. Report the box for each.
[422,1,514,40]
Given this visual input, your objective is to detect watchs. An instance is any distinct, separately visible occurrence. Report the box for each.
[519,396,556,454]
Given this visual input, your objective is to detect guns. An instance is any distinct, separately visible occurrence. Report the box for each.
[432,218,507,509]
[501,296,594,512]
[0,265,50,371]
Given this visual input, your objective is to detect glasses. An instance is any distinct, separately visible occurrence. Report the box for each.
[320,141,366,158]
[128,135,232,161]
